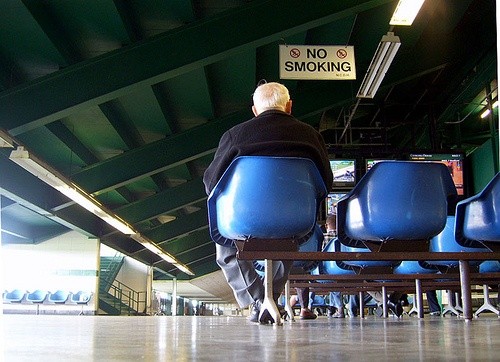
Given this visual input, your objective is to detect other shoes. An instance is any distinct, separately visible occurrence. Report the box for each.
[430,311,440,316]
[332,307,344,318]
[374,305,383,318]
[278,307,287,315]
[345,303,354,318]
[389,301,403,317]
[300,309,317,319]
[250,298,264,322]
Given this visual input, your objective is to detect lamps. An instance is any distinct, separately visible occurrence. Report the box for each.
[0,127,195,277]
[355,0,500,120]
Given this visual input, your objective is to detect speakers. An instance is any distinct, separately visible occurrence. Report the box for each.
[329,159,356,187]
[365,159,397,174]
[410,154,468,199]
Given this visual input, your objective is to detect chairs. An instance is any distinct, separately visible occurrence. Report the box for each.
[26,289,51,315]
[49,289,73,304]
[204,153,500,326]
[4,287,29,302]
[70,290,94,316]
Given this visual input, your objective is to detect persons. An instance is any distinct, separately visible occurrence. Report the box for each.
[274,216,442,319]
[203,81,332,322]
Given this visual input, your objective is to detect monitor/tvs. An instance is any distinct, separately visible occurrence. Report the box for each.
[324,191,352,218]
